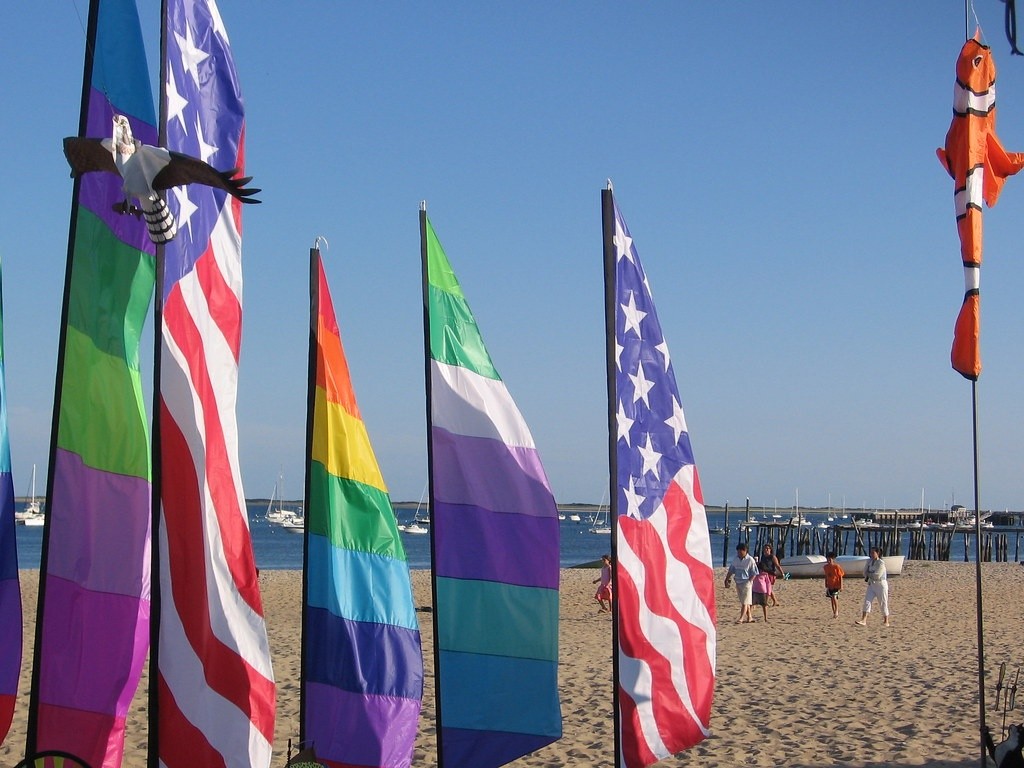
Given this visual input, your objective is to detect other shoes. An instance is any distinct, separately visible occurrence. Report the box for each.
[598,606,613,616]
[735,618,754,624]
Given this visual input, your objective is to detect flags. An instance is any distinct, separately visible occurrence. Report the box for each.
[0,266,24,746]
[601,178,718,768]
[299,250,423,767]
[420,209,563,768]
[148,1,277,768]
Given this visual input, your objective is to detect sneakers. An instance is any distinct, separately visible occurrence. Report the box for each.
[881,622,889,627]
[855,619,866,626]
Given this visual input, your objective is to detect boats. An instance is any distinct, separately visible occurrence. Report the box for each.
[280,474,304,533]
[559,514,566,520]
[827,493,834,522]
[265,471,297,522]
[838,494,849,519]
[816,521,831,528]
[14,463,45,527]
[397,478,429,534]
[762,485,995,529]
[570,514,582,521]
[780,553,906,577]
[586,481,611,534]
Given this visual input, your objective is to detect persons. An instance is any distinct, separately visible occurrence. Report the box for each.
[823,552,845,619]
[25,0,161,767]
[854,547,890,626]
[592,555,614,615]
[759,544,789,608]
[750,562,774,622]
[725,544,759,623]
